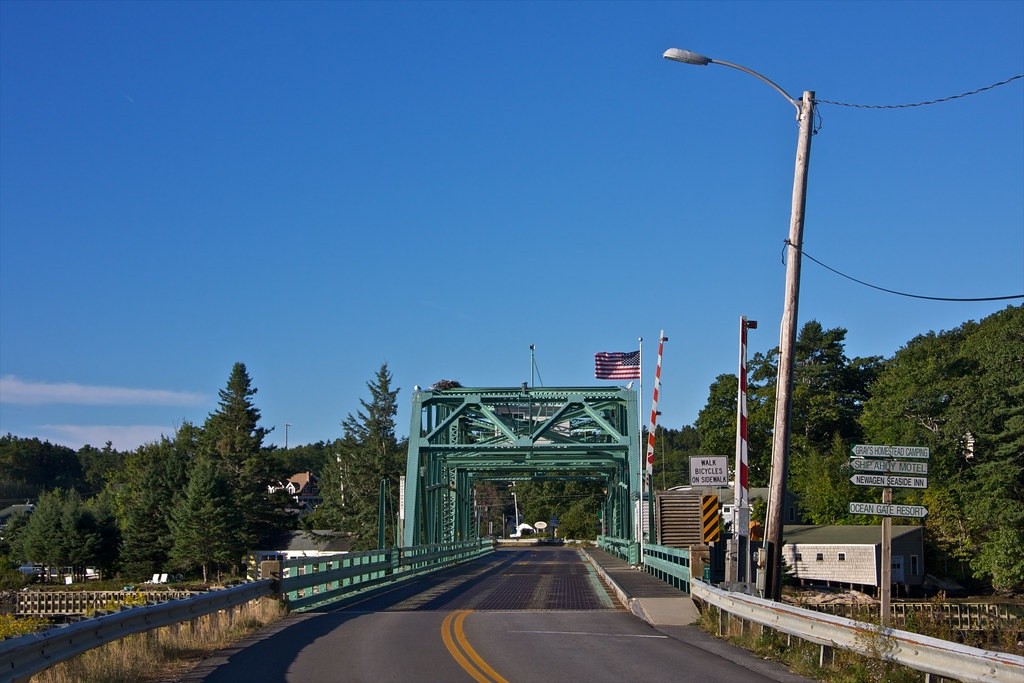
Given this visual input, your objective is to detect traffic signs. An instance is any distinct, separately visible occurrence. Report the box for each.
[851,443,930,489]
[848,503,929,520]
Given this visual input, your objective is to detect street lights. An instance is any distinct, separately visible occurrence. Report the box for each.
[662,46,819,605]
[285,423,292,449]
[511,493,518,527]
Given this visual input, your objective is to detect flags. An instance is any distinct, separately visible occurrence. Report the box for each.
[595,350,640,379]
